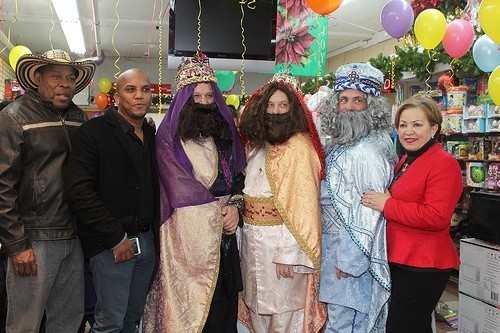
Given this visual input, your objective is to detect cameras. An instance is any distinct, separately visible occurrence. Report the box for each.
[115,237,141,260]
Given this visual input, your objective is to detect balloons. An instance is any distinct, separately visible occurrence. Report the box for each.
[414,8,447,51]
[305,0,344,17]
[10,45,32,72]
[442,19,475,59]
[380,0,414,42]
[213,69,236,93]
[478,0,500,44]
[226,94,241,110]
[98,77,112,95]
[91,112,99,118]
[488,66,500,108]
[473,34,500,75]
[96,94,107,110]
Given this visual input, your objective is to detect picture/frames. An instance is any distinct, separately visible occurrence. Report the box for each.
[404,81,429,100]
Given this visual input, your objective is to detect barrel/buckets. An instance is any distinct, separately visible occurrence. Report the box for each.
[439,86,468,134]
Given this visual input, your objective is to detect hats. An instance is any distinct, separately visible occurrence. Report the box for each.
[16,49,97,95]
[333,63,384,98]
[268,68,303,98]
[175,50,218,91]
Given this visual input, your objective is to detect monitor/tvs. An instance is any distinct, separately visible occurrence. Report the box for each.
[168,0,277,62]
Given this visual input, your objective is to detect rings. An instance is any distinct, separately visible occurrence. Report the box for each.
[368,199,370,205]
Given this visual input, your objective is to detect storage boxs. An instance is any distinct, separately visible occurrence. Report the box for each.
[457,238,500,333]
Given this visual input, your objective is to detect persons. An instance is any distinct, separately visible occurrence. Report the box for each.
[318,64,399,333]
[155,54,247,333]
[64,68,160,333]
[237,74,328,333]
[361,94,464,333]
[0,49,87,333]
